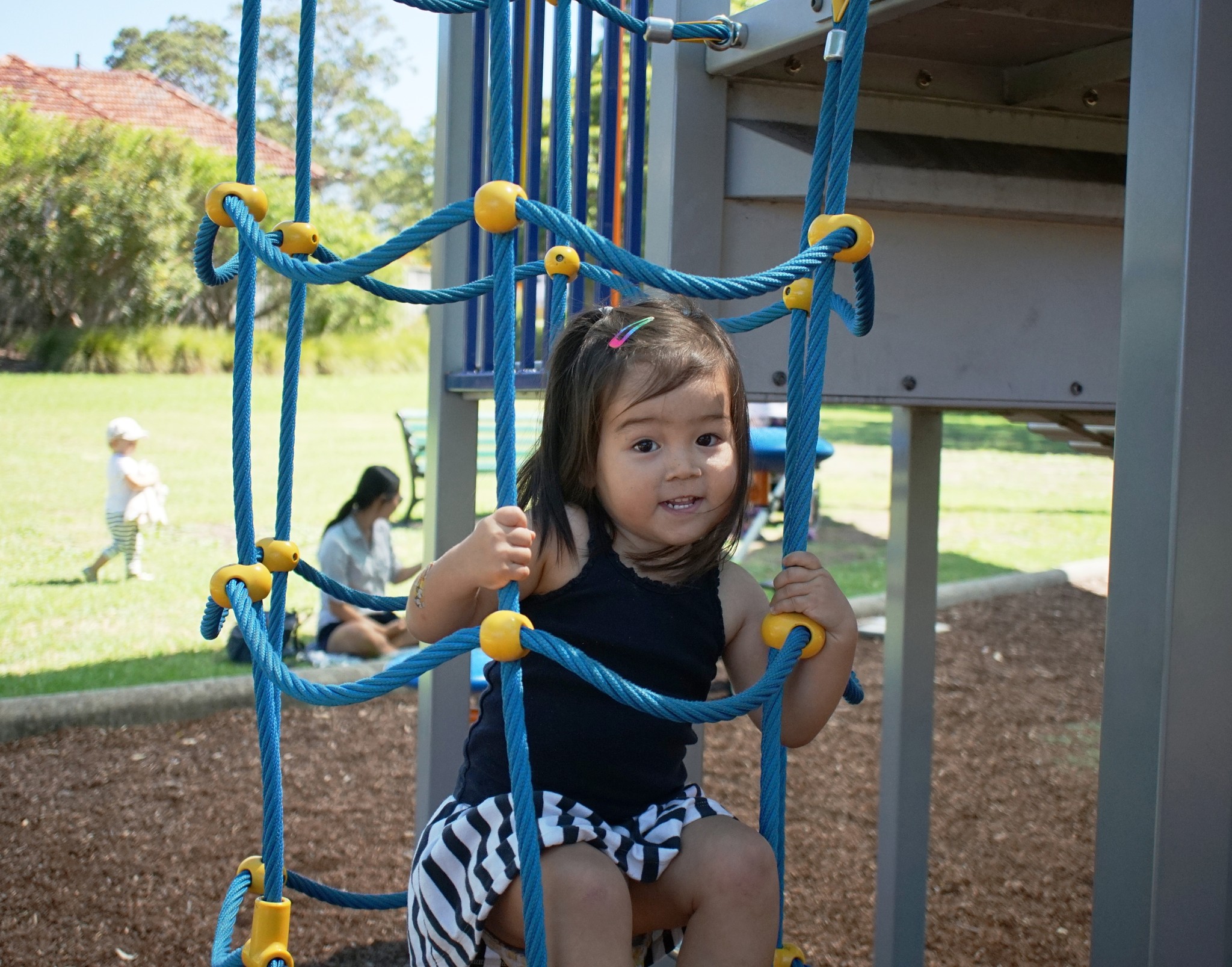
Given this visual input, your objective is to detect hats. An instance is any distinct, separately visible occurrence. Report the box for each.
[106,417,147,442]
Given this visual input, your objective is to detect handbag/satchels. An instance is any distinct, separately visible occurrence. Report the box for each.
[227,612,298,662]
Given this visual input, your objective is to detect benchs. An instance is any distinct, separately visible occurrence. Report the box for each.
[392,408,545,532]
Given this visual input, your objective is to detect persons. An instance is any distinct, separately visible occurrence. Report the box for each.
[79,418,160,587]
[409,302,855,967]
[316,466,425,654]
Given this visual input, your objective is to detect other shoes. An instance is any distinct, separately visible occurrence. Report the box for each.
[128,571,155,583]
[84,567,98,584]
